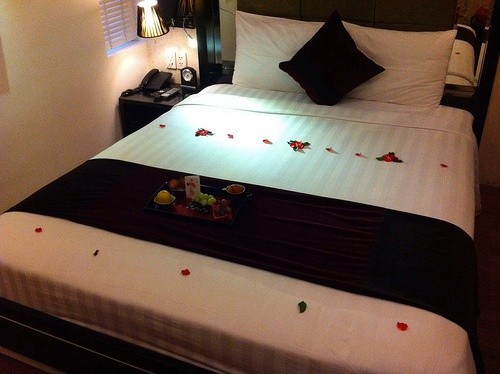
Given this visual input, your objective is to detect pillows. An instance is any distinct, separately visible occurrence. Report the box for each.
[342,21,458,107]
[231,11,325,94]
[279,10,385,106]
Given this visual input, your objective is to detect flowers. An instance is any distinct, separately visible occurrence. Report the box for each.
[159,123,403,164]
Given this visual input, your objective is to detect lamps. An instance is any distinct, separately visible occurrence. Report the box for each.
[137,0,170,38]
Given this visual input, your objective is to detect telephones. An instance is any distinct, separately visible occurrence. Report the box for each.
[140,69,172,91]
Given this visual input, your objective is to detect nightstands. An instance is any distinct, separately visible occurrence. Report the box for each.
[119,83,182,139]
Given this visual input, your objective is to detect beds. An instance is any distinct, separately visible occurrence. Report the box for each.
[0,0,482,374]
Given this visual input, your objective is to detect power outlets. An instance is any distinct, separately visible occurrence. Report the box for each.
[176,51,187,70]
[166,52,176,70]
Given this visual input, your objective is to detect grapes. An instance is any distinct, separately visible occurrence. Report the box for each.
[186,192,217,214]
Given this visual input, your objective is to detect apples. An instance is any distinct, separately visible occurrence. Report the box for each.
[156,190,171,203]
[169,177,185,189]
[230,184,242,192]
[212,198,227,216]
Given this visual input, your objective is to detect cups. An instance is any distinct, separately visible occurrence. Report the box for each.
[153,195,177,210]
[212,203,226,215]
[226,184,246,199]
